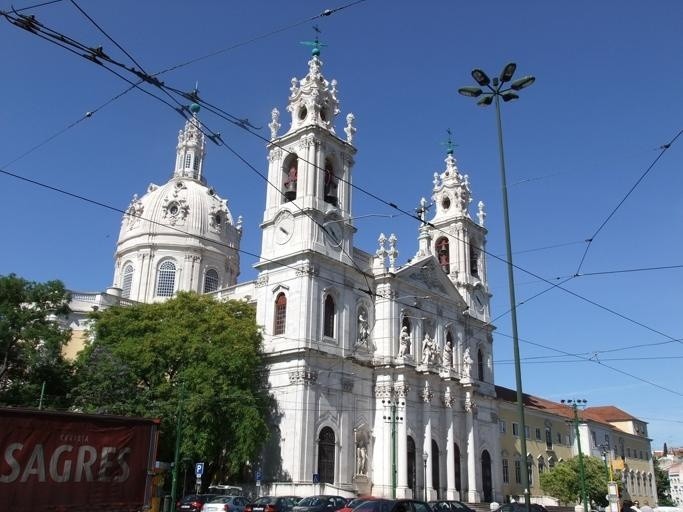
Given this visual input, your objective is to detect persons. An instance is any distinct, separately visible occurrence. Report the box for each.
[464,349,473,377]
[354,438,368,478]
[422,334,432,364]
[620,499,653,512]
[358,311,369,342]
[399,325,411,358]
[443,342,451,368]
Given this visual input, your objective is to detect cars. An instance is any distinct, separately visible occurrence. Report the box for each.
[198,495,250,512]
[491,502,548,512]
[243,497,294,512]
[334,497,475,512]
[176,494,217,512]
[291,496,347,512]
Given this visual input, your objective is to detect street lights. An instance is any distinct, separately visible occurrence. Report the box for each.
[380,387,408,500]
[598,442,609,485]
[181,457,192,499]
[454,60,534,511]
[421,452,428,504]
[560,398,592,512]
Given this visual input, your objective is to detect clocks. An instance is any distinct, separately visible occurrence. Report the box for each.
[473,289,485,312]
[322,219,343,247]
[272,219,294,245]
